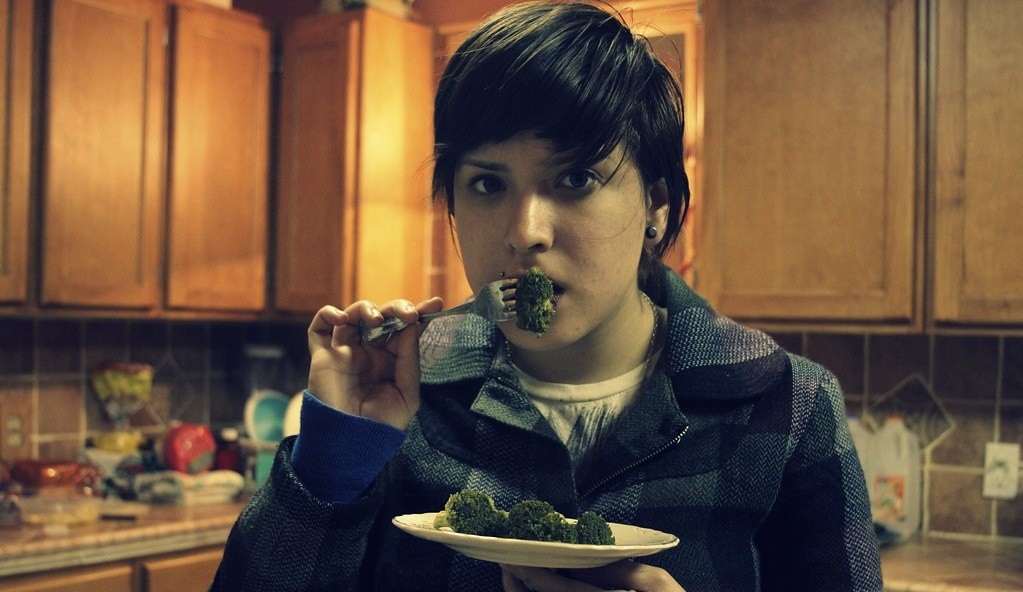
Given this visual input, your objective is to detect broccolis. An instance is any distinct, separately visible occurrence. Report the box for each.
[434,493,615,545]
[515,266,558,334]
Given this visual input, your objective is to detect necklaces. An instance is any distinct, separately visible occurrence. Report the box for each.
[506,288,659,393]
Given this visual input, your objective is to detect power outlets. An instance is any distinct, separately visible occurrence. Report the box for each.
[982,442,1019,500]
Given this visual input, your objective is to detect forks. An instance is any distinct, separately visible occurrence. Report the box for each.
[358,278,519,343]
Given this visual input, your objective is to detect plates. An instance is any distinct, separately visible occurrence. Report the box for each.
[392,510,680,569]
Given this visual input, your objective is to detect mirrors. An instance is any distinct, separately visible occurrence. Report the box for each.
[435,0,704,326]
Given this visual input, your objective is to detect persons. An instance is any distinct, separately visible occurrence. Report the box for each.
[205,1,884,592]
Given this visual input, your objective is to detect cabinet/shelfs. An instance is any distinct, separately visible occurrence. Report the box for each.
[272,8,429,316]
[0,508,223,592]
[0,1,269,323]
[698,1,1023,336]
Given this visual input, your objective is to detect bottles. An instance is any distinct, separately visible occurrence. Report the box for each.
[846,414,875,523]
[873,415,924,536]
